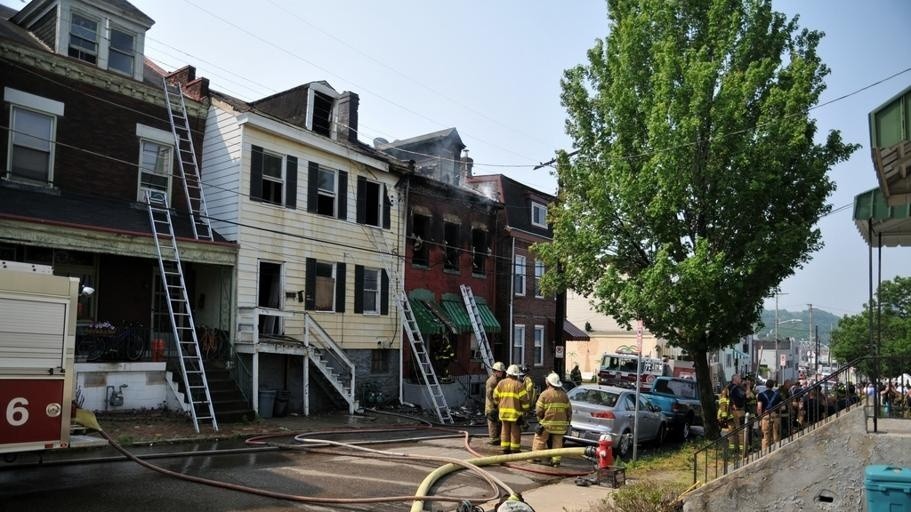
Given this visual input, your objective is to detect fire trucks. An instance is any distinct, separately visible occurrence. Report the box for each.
[599,352,665,395]
[0,257,97,455]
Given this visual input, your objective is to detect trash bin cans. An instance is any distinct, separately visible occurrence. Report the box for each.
[864,465,911,512]
[258,389,291,418]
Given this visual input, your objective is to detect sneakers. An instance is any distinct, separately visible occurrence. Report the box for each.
[530,458,542,465]
[488,439,501,445]
[503,449,522,454]
[551,462,560,468]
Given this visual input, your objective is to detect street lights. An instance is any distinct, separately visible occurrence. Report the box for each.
[774,319,803,388]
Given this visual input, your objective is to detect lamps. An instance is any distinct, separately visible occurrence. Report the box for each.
[78,282,97,298]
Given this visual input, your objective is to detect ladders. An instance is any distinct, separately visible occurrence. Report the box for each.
[460,284,496,377]
[145,189,220,436]
[393,290,455,425]
[162,77,214,242]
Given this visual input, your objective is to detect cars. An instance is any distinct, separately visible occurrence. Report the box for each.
[564,376,727,461]
[758,374,768,384]
[755,386,776,401]
[797,366,838,394]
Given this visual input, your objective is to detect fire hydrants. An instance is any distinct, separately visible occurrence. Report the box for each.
[593,431,616,471]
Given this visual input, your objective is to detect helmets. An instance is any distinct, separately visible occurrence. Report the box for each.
[492,361,507,372]
[505,364,522,376]
[544,372,563,388]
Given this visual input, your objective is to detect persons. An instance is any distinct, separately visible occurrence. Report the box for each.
[485,361,507,444]
[571,365,583,386]
[525,372,573,467]
[493,364,530,454]
[519,364,539,431]
[717,374,911,458]
